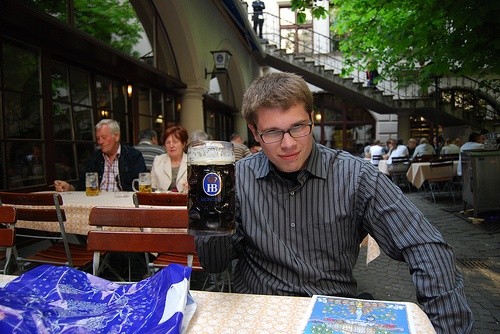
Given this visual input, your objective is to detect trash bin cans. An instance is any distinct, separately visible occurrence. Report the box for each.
[460,148,500,210]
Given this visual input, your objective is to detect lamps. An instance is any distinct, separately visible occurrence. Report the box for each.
[204,47,233,79]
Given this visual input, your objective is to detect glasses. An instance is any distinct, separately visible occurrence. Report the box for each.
[255,118,313,144]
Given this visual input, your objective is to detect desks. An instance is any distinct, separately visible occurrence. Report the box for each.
[405,160,459,206]
[377,159,413,178]
[0,274,437,334]
[2,191,187,282]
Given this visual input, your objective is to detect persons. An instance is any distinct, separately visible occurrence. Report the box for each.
[132,129,166,173]
[366,63,379,91]
[457,132,485,177]
[146,126,190,192]
[364,140,374,160]
[252,0,266,38]
[367,140,387,166]
[191,130,207,142]
[228,133,250,162]
[55,118,147,279]
[195,71,473,334]
[388,139,409,165]
[431,136,441,153]
[440,136,459,160]
[412,137,437,160]
[382,138,395,160]
[406,138,416,156]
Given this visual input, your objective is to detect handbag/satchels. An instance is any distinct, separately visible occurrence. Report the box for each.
[0,264,197,334]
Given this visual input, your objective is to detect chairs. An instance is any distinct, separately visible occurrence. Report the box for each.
[133,191,206,278]
[85,204,197,284]
[427,157,457,208]
[440,153,460,192]
[420,154,440,193]
[372,154,383,167]
[0,191,111,277]
[388,156,414,194]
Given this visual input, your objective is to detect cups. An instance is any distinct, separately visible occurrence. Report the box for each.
[187,140,237,237]
[86,172,98,196]
[132,173,152,193]
